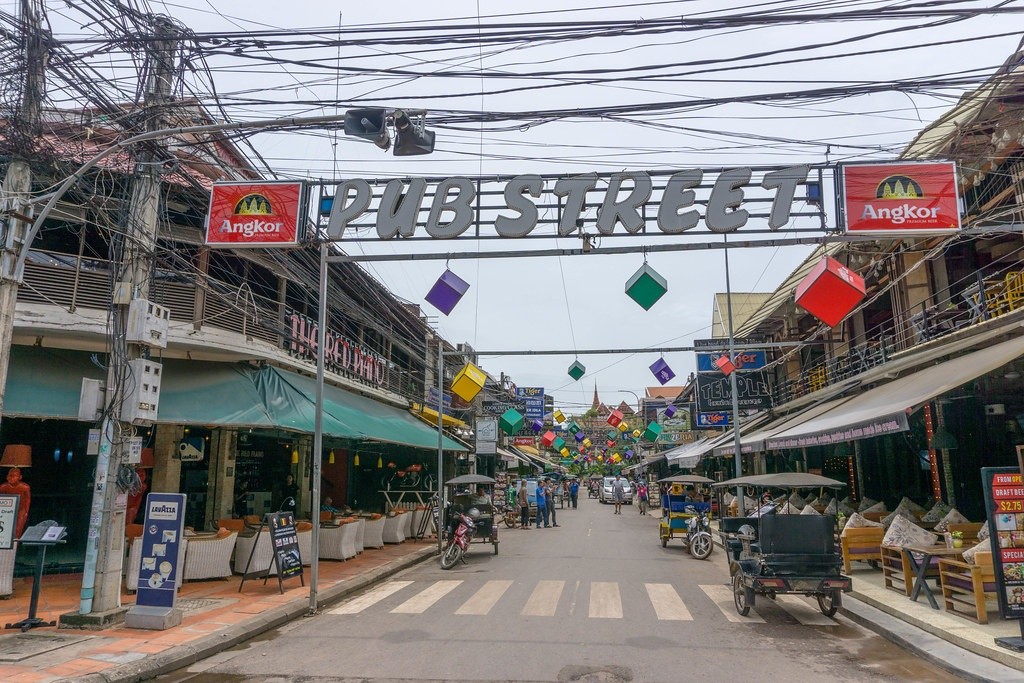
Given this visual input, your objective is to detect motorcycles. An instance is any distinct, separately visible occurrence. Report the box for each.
[439,508,485,569]
[710,472,853,616]
[654,475,716,561]
[443,473,500,555]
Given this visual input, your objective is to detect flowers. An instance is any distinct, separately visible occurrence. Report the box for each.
[953,529,965,539]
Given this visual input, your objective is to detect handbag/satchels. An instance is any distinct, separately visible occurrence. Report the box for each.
[641,495,647,501]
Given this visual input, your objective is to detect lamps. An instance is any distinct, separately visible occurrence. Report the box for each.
[291,438,299,463]
[329,445,334,463]
[354,448,360,466]
[0,444,32,468]
[454,424,475,436]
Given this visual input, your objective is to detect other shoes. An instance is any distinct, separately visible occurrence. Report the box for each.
[521,527,532,530]
[544,524,552,528]
[640,511,642,514]
[643,513,645,515]
[537,525,541,528]
[553,524,561,527]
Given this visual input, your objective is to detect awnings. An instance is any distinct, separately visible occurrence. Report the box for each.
[621,445,682,474]
[5,344,273,429]
[766,334,1024,451]
[664,437,707,467]
[496,447,567,471]
[257,365,472,452]
[713,394,857,456]
[678,416,770,468]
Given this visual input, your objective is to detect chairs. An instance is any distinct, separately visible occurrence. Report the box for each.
[123,504,434,591]
[786,269,1023,399]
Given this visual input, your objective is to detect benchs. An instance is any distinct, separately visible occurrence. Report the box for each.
[671,502,711,519]
[454,495,471,507]
[662,495,686,512]
[378,452,383,468]
[725,505,1004,625]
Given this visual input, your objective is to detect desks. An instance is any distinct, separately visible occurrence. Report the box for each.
[853,340,892,371]
[912,308,971,341]
[976,277,1015,317]
[902,543,978,609]
[785,378,809,398]
[828,355,850,382]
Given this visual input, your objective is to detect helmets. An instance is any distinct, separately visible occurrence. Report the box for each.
[739,524,756,536]
[685,505,695,514]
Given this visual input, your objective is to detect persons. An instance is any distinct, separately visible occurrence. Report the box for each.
[761,490,772,505]
[612,475,624,514]
[279,474,300,512]
[568,479,579,509]
[630,480,647,515]
[234,479,249,514]
[508,481,520,528]
[685,485,704,502]
[588,479,599,495]
[520,479,530,530]
[536,477,561,528]
[661,484,667,495]
[477,486,498,513]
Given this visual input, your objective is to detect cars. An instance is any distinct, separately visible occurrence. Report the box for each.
[599,477,633,505]
[507,480,544,520]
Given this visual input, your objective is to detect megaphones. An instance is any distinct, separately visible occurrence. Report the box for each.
[344,108,391,149]
[393,109,435,156]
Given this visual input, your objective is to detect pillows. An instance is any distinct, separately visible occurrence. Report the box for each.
[780,492,991,567]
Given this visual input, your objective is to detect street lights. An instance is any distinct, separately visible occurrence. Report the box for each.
[617,389,642,476]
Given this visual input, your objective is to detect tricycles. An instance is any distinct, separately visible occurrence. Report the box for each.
[587,489,600,499]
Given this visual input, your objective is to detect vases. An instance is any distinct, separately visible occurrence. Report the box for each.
[954,537,963,547]
